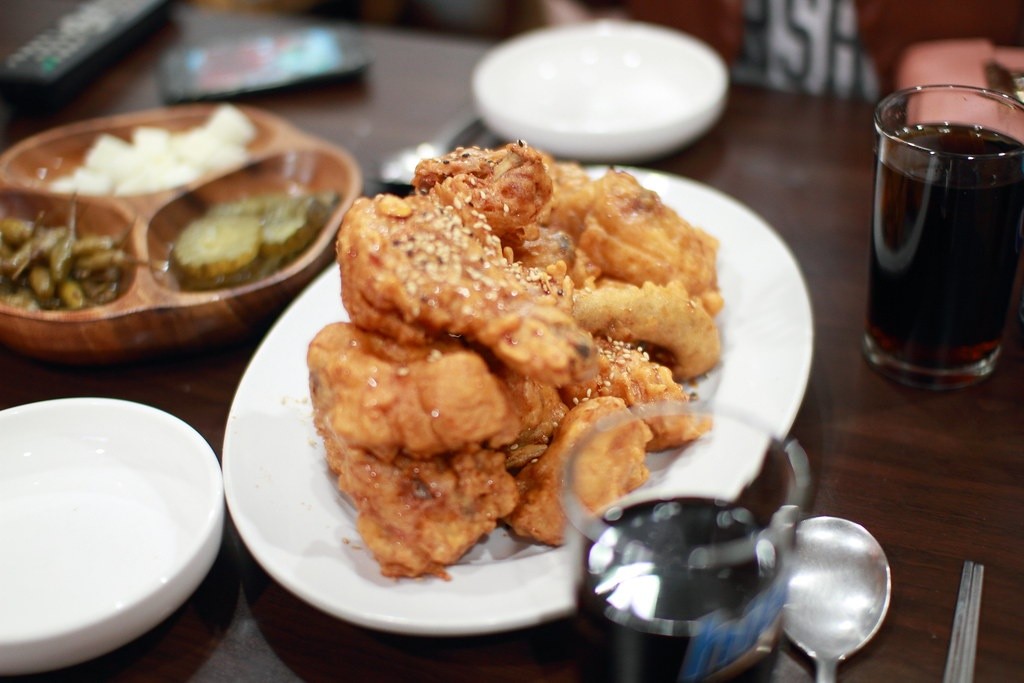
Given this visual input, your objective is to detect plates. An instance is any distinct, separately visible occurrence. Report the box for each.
[0,103,364,357]
[221,167,815,632]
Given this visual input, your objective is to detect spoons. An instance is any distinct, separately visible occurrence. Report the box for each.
[778,516,891,683]
[376,122,480,184]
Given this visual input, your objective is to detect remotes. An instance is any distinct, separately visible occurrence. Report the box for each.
[0,0,172,102]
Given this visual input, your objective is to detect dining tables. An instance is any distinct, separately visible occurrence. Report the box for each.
[0,1,1024,682]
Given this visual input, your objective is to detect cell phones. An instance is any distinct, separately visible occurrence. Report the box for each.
[163,20,372,106]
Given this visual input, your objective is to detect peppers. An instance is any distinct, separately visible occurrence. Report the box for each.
[0,192,169,312]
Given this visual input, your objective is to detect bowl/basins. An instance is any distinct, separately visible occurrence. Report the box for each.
[474,20,732,163]
[0,398,225,675]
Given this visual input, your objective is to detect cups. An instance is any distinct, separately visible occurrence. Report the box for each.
[557,404,805,683]
[864,85,1024,392]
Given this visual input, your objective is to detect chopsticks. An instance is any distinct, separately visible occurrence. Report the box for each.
[943,562,984,683]
